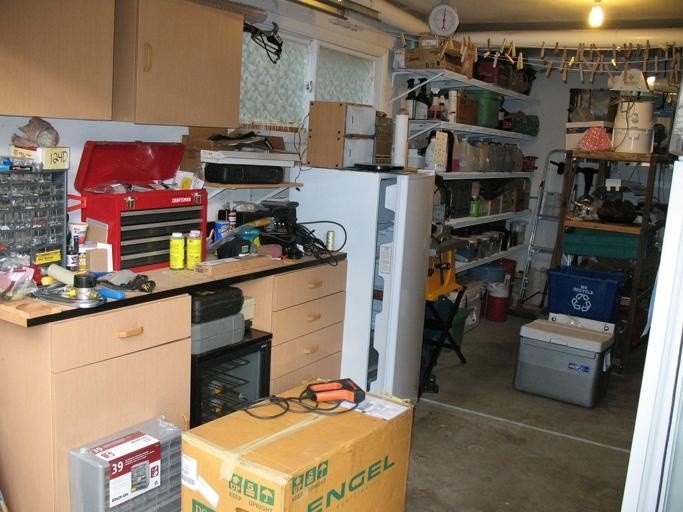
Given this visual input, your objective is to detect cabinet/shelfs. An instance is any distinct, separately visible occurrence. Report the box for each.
[0,1,244,130]
[227,259,348,398]
[0,293,192,510]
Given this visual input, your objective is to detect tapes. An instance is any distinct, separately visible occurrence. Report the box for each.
[41,276,54,287]
[74,273,97,287]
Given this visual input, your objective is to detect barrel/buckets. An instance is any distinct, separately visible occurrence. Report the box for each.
[425,131,523,172]
[467,88,505,127]
[485,288,509,322]
[475,266,506,281]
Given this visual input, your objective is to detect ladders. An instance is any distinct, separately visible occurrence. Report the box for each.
[517,149,578,309]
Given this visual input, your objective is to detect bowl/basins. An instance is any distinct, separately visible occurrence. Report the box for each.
[467,263,510,284]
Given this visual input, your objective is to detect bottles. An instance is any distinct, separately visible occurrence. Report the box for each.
[425,134,524,173]
[170,231,184,270]
[404,78,457,120]
[469,195,480,217]
[185,230,202,269]
[207,380,245,416]
[67,245,87,272]
[213,210,238,256]
[509,220,529,246]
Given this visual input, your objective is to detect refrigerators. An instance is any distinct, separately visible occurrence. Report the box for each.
[288,164,435,410]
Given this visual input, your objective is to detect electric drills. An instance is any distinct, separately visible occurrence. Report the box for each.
[287,378,365,403]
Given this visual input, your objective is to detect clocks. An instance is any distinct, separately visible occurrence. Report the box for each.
[427,4,459,37]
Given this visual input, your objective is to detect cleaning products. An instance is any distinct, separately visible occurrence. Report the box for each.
[429,86,448,121]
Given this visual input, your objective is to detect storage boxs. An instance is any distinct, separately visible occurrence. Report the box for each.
[512,317,616,408]
[178,376,415,510]
[72,140,208,274]
